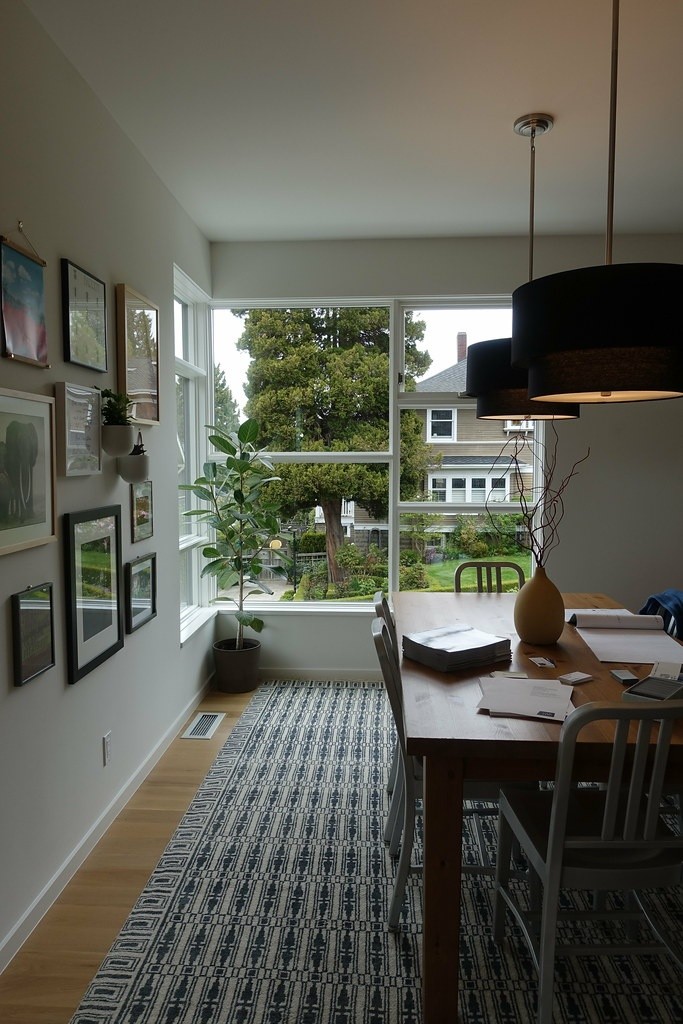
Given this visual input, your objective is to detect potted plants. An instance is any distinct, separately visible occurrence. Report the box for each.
[177,419,282,692]
[117,444,150,484]
[94,385,138,458]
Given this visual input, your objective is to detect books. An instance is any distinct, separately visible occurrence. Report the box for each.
[476,676,577,722]
[401,618,514,676]
[564,609,683,664]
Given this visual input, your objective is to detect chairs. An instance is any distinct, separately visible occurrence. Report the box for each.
[371,562,683,1024]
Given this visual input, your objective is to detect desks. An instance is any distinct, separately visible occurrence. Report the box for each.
[391,590,683,1024]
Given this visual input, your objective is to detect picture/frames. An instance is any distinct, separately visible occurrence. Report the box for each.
[125,552,157,634]
[0,387,59,555]
[10,582,56,687]
[131,481,154,544]
[60,257,108,373]
[63,504,125,686]
[117,284,161,425]
[54,382,103,477]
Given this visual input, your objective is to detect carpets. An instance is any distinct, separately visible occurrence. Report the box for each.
[69,681,683,1024]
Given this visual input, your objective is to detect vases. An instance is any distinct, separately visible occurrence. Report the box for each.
[514,566,565,648]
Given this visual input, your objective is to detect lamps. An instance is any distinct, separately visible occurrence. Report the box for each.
[513,0,683,405]
[468,114,579,420]
